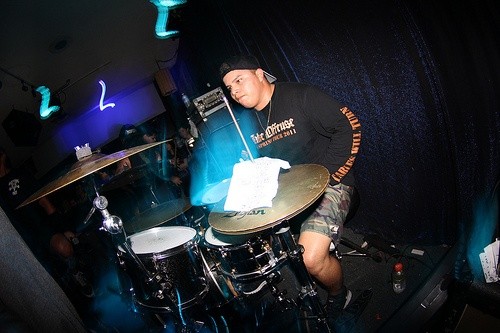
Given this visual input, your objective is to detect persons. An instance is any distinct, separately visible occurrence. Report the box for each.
[0,125,198,315]
[219,53,361,324]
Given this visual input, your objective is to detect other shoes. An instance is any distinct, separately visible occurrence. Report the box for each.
[81,283,94,297]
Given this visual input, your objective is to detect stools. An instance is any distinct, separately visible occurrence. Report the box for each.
[289,185,382,264]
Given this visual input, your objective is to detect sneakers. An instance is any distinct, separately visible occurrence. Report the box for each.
[323,286,351,320]
[242,269,280,294]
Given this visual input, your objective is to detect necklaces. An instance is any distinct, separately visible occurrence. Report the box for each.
[254,87,274,140]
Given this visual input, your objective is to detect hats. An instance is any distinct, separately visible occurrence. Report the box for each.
[219,55,277,83]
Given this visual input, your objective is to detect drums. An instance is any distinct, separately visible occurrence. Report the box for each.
[119,225,210,313]
[204,225,294,281]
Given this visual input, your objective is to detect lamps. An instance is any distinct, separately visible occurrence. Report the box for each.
[154,49,179,96]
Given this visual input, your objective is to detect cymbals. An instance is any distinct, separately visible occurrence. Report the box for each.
[209,163,331,235]
[123,198,193,234]
[16,139,174,210]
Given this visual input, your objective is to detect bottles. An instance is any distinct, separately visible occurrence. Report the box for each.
[392,264,406,293]
[182,93,191,108]
